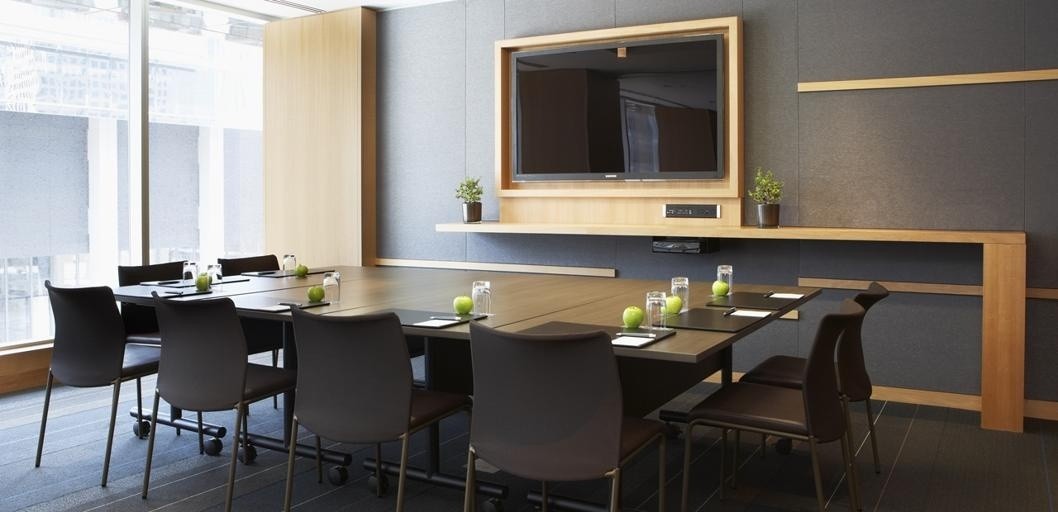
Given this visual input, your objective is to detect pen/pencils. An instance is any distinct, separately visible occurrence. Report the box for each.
[278,303,302,306]
[723,307,735,316]
[165,291,183,294]
[616,333,656,338]
[158,281,180,284]
[763,291,774,298]
[259,271,275,274]
[430,317,461,321]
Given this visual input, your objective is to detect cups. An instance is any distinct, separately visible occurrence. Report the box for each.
[645,291,667,333]
[182,262,198,286]
[323,272,341,305]
[671,277,689,313]
[717,265,734,295]
[472,280,492,316]
[282,254,297,276]
[208,264,223,289]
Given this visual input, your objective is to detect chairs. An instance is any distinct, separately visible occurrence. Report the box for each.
[117,261,185,347]
[217,255,280,408]
[737,280,889,491]
[35,280,161,487]
[142,291,283,512]
[283,307,470,512]
[463,321,670,511]
[679,297,867,512]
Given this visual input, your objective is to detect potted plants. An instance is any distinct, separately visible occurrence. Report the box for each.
[455,178,483,223]
[747,167,784,228]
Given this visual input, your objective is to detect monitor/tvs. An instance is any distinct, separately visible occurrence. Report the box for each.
[509,32,726,181]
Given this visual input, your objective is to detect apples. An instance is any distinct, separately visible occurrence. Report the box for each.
[623,306,643,328]
[660,295,682,314]
[296,264,308,277]
[712,280,730,296]
[197,272,211,291]
[307,285,325,302]
[453,293,472,315]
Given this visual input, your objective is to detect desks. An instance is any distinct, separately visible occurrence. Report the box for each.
[113,266,822,417]
